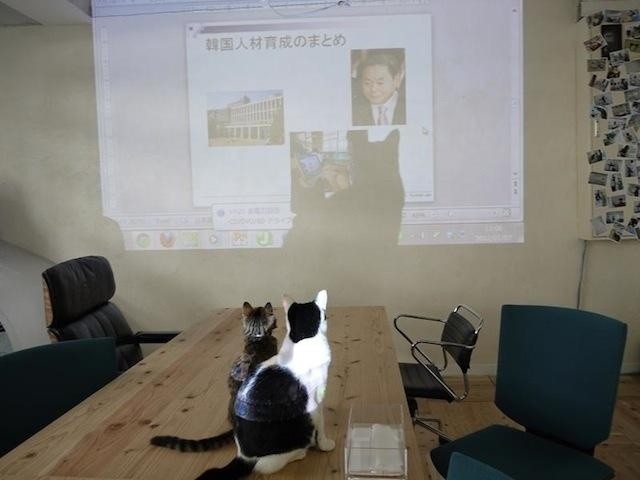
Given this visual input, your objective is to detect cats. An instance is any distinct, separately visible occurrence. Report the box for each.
[281,130,404,306]
[195,289,336,480]
[149,302,277,453]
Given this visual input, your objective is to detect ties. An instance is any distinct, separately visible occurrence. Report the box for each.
[378,105,388,125]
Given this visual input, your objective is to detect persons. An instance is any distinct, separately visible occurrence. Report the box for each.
[352,53,407,125]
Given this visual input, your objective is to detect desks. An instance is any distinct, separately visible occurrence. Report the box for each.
[0,305,427,480]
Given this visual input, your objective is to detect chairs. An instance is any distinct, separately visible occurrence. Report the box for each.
[430,304,627,480]
[0,336,119,457]
[40,255,182,375]
[393,304,485,442]
